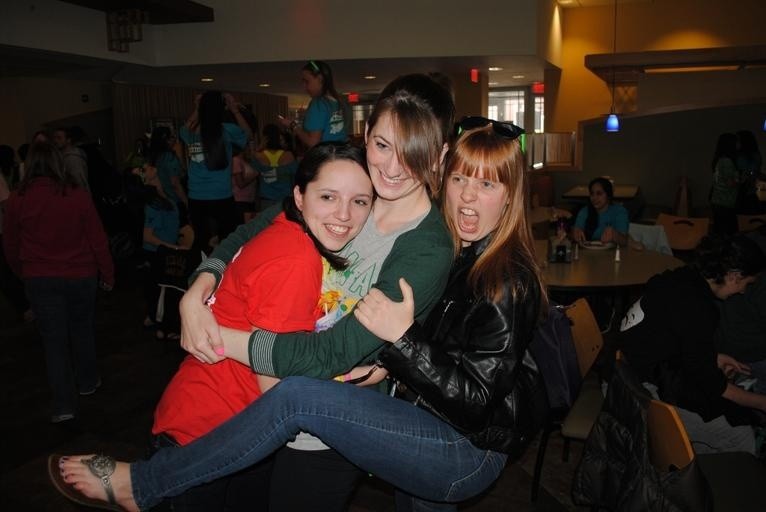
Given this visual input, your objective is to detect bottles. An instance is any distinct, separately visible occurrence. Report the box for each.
[614,244,621,262]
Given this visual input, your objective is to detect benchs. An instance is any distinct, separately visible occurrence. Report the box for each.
[632,177,690,225]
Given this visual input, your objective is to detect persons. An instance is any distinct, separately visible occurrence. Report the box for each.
[179,73,454,512]
[48,114,549,510]
[151,139,376,512]
[733,130,763,212]
[1,59,353,394]
[616,233,765,454]
[704,129,739,244]
[573,176,630,244]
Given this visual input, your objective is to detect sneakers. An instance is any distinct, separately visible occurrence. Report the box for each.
[51,413,74,423]
[79,375,101,395]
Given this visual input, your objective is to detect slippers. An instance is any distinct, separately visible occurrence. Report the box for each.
[46,452,142,511]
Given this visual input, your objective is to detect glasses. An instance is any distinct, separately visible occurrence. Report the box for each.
[453,116,526,140]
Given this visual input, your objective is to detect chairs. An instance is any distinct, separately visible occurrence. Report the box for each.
[643,396,699,473]
[653,214,712,259]
[531,299,606,507]
[736,212,765,230]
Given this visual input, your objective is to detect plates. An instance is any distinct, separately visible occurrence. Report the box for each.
[582,240,614,250]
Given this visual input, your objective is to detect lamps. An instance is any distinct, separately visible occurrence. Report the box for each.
[468,64,480,87]
[531,71,544,94]
[606,1,621,132]
[347,91,359,103]
